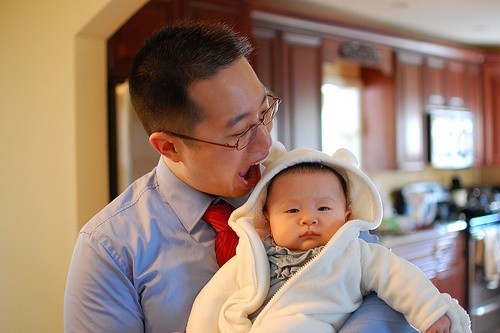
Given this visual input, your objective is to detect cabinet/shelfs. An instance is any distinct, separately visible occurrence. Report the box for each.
[106,0,500,171]
[386,230,468,312]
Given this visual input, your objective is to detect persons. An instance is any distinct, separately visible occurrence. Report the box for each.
[64,19,420,333]
[185,139,471,333]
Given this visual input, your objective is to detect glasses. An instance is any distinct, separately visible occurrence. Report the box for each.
[160,93,283,151]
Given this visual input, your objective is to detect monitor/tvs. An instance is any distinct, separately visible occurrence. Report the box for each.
[427,109,474,171]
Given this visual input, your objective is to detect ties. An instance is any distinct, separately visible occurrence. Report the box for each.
[202,201,240,267]
